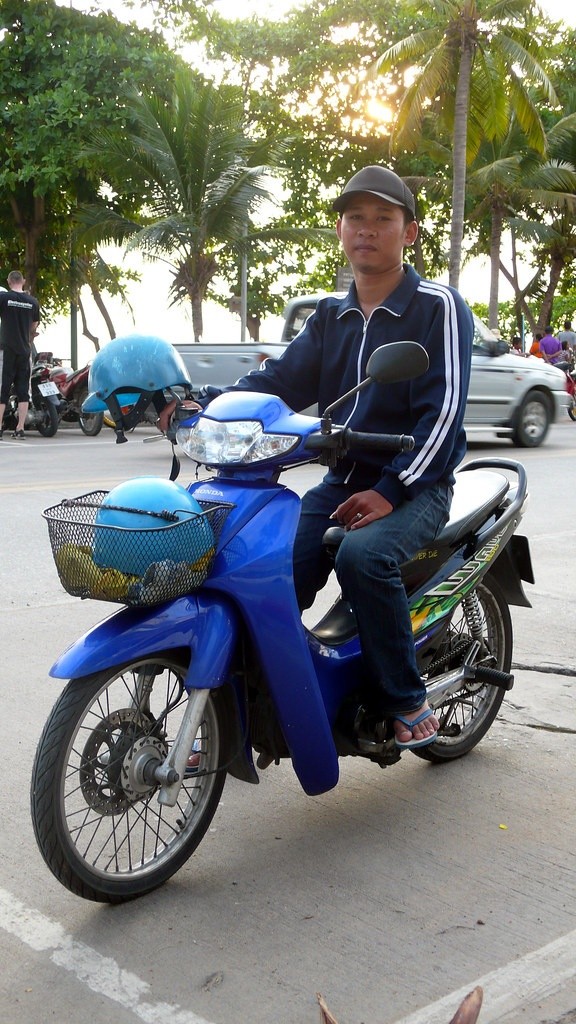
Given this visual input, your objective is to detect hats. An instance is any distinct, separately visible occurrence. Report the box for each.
[330,164,416,222]
[545,326,554,331]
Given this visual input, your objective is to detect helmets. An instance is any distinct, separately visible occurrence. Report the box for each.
[80,335,194,414]
[90,475,215,579]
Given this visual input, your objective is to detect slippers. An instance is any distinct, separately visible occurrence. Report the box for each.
[184,739,201,773]
[392,707,439,750]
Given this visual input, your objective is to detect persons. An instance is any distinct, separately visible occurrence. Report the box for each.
[0,271,41,440]
[490,321,576,366]
[158,167,476,773]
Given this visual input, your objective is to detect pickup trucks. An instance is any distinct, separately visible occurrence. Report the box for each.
[150,286,573,447]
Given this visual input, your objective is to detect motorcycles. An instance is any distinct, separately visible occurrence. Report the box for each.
[3,358,67,438]
[49,358,106,437]
[29,340,540,906]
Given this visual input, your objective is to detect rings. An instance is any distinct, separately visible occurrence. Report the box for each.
[357,513,363,521]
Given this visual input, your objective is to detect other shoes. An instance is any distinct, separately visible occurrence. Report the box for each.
[0,430,3,440]
[9,429,26,440]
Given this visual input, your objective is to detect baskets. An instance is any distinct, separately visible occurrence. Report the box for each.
[39,487,238,611]
